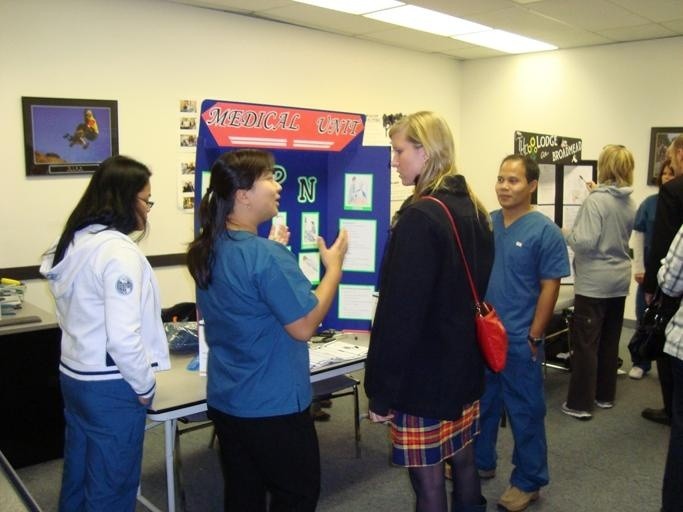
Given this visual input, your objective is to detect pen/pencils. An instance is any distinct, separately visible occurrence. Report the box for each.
[579,174,588,184]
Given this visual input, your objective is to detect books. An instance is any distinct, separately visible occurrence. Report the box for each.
[0,286,24,315]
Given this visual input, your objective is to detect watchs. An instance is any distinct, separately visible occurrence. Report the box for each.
[527,335,543,346]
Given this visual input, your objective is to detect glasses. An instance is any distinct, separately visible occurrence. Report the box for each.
[138,197,154,209]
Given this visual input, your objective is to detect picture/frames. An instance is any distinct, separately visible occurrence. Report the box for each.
[22,96,119,176]
[647,127,682,185]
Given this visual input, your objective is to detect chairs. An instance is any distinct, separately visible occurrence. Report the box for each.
[136,331,374,512]
[162,302,217,512]
[310,374,361,460]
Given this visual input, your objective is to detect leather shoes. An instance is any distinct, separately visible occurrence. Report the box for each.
[642,407,667,423]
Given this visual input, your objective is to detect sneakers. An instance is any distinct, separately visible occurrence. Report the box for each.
[496,486,540,511]
[562,402,592,419]
[442,460,495,477]
[595,398,613,409]
[630,365,642,378]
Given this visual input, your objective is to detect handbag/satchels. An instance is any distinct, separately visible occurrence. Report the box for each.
[419,194,506,371]
[627,282,681,362]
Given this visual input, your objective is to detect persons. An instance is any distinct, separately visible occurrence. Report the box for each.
[363,110,495,511]
[656,223,683,512]
[560,144,637,419]
[627,159,674,380]
[39,155,171,512]
[187,147,349,512]
[74,108,98,142]
[641,133,683,426]
[487,154,570,512]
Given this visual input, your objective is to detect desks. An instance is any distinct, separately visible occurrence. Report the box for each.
[0,301,64,469]
